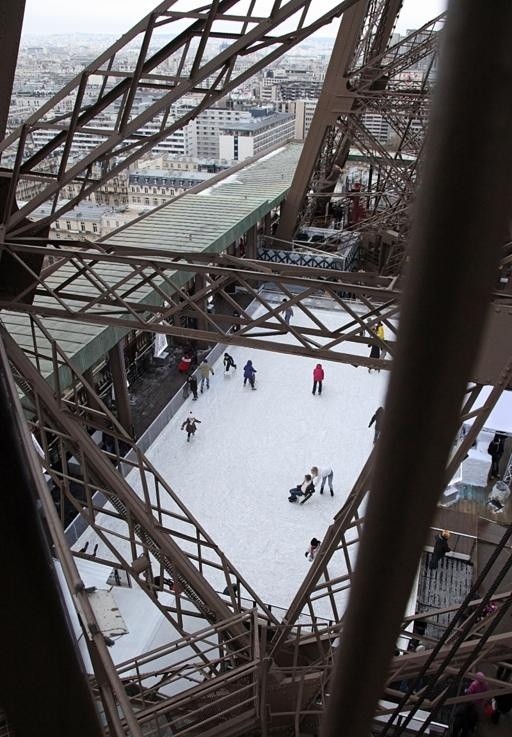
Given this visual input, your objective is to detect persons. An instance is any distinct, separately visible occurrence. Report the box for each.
[187,353,257,400]
[312,363,324,395]
[368,405,384,444]
[429,530,451,569]
[305,537,322,562]
[358,318,385,372]
[282,299,293,325]
[288,466,335,506]
[181,414,201,442]
[453,672,496,737]
